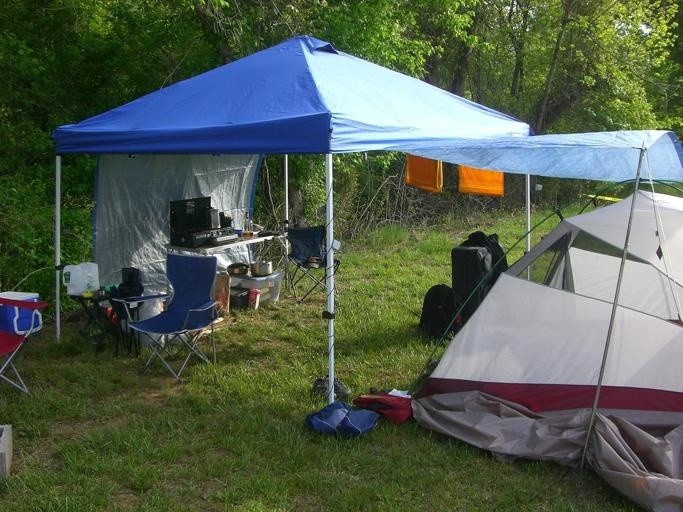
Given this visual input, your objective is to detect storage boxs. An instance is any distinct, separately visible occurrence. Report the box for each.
[0,291,43,336]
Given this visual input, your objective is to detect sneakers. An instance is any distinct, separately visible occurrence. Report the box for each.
[315,375,351,398]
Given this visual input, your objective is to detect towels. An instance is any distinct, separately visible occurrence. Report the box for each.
[406,154,443,195]
[457,164,505,197]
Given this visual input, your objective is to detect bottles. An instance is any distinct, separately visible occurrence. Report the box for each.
[209,209,221,229]
[243,212,253,240]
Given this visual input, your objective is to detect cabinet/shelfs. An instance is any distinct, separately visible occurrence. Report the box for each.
[229,269,284,311]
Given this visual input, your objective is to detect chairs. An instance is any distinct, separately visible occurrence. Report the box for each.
[112,253,224,383]
[0,297,49,398]
[284,225,341,304]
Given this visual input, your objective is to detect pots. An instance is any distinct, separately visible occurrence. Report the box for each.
[227,262,248,275]
[249,261,273,277]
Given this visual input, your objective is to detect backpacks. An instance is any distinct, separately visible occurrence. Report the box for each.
[418,284,462,336]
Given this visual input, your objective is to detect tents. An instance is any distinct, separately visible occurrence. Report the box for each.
[408,177,682,512]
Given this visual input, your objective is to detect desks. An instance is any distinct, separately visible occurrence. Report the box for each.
[70,288,168,358]
[163,230,288,343]
